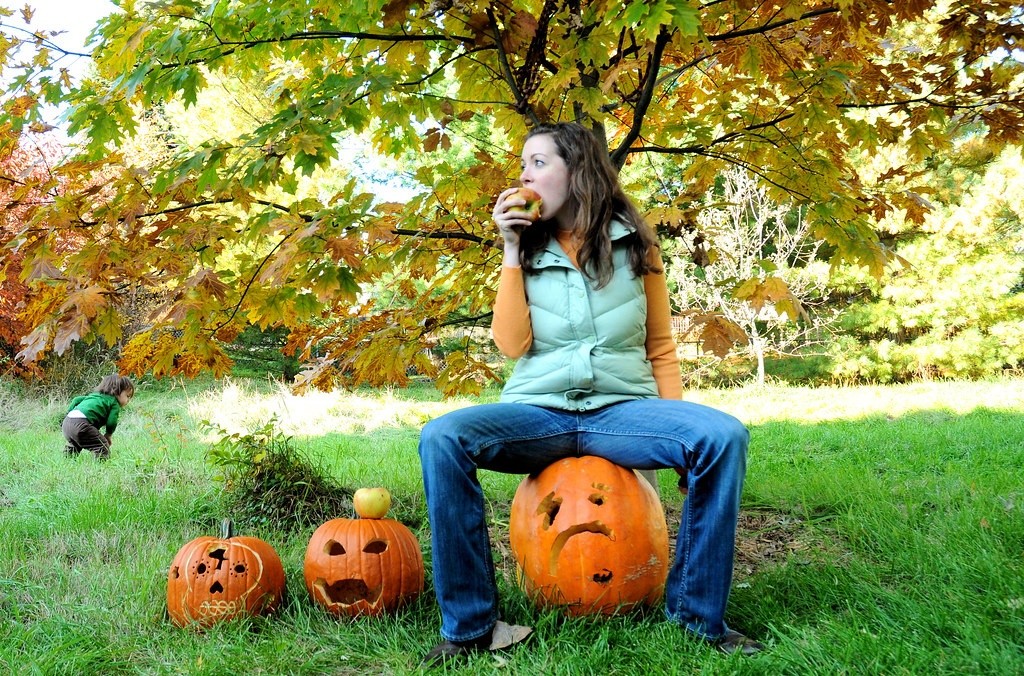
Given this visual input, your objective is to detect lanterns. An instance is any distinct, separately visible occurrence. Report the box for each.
[168,519,287,632]
[509,455,669,616]
[306,519,424,615]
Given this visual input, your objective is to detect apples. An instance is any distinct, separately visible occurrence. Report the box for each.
[353,487,391,519]
[504,187,542,222]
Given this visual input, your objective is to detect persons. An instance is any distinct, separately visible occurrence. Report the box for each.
[419,121,754,665]
[62,374,134,459]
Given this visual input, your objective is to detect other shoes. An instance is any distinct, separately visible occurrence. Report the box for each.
[424,624,495,672]
[713,629,760,656]
